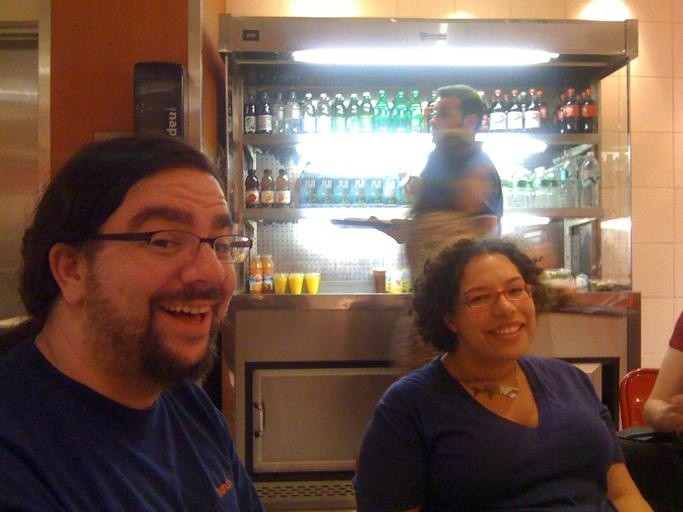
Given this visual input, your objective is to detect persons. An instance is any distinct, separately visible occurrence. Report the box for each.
[378,84,504,375]
[391,129,576,376]
[1,134,263,512]
[354,237,655,512]
[642,309,682,432]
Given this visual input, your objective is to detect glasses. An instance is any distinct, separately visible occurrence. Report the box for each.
[456,284,535,309]
[89,230,252,264]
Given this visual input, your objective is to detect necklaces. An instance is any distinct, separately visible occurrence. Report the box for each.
[448,352,521,399]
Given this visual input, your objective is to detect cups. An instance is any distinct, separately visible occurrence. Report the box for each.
[305,272,319,294]
[287,272,304,295]
[271,272,287,293]
[372,268,412,293]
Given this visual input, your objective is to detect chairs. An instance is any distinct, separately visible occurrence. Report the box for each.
[618,367,659,430]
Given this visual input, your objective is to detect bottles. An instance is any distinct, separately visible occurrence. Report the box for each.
[299,151,601,207]
[261,169,274,207]
[248,254,274,293]
[243,169,259,207]
[275,168,291,207]
[243,82,599,133]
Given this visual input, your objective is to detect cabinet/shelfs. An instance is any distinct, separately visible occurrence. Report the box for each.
[230,64,603,221]
